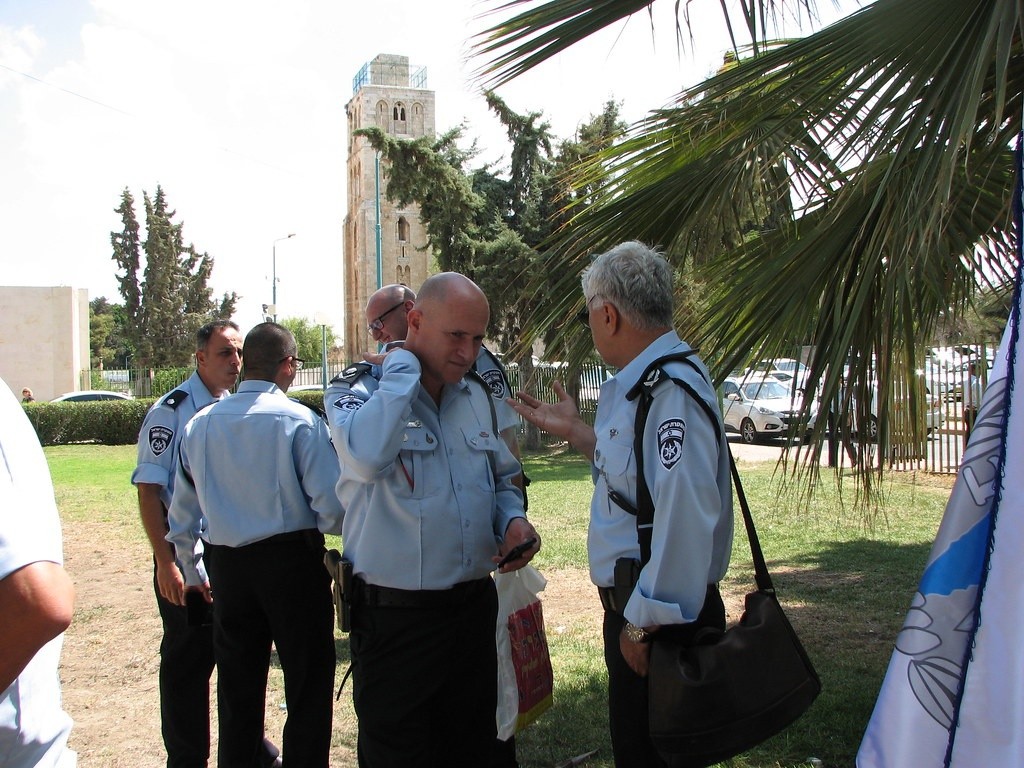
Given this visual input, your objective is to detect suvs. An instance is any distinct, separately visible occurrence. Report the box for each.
[712,374,822,444]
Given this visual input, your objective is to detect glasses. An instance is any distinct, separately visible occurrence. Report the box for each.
[366,301,404,334]
[577,294,612,321]
[279,355,306,370]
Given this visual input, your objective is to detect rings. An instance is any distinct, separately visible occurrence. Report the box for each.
[531,412,533,415]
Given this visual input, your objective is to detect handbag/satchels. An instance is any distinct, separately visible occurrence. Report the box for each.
[624,347,823,768]
[493,562,553,741]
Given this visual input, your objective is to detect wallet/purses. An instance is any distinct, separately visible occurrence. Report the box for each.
[185,590,214,626]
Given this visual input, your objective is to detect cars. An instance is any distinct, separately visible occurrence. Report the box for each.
[746,371,818,399]
[924,346,964,369]
[47,389,136,403]
[867,362,951,394]
[823,351,877,379]
[939,356,995,402]
[493,353,540,368]
[949,344,999,358]
[744,358,823,397]
[817,380,946,441]
[537,361,615,411]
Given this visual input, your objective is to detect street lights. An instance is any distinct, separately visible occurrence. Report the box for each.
[272,232,297,325]
[125,353,135,370]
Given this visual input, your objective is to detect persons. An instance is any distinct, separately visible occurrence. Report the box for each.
[507,239,735,768]
[164,323,345,768]
[0,378,79,768]
[817,376,859,467]
[367,282,531,768]
[961,363,982,464]
[22,388,35,402]
[324,272,541,768]
[132,321,243,768]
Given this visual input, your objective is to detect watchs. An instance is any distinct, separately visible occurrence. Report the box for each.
[624,623,656,644]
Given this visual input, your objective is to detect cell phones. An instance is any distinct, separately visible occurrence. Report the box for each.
[498,538,537,569]
